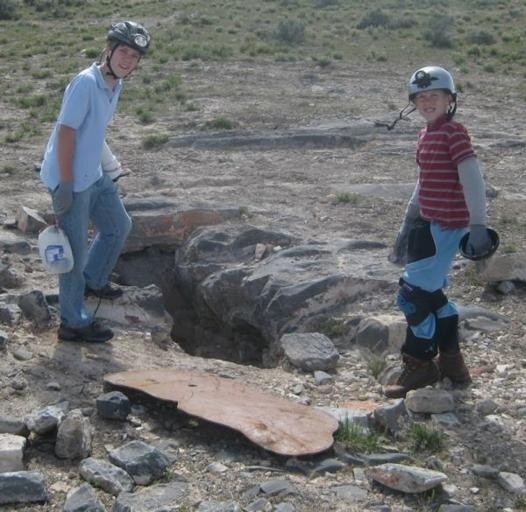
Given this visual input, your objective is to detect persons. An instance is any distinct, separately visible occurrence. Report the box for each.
[38,20,152,346]
[382,62,493,400]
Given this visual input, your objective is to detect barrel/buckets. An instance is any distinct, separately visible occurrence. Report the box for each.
[37,224,75,274]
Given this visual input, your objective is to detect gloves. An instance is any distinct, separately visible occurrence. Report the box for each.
[53,181,73,218]
[392,226,409,268]
[465,223,494,257]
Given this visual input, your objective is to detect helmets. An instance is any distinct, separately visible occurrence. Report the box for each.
[106,20,151,55]
[407,66,456,99]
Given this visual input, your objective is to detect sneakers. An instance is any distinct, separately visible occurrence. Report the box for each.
[385,352,443,398]
[58,320,114,343]
[84,282,124,300]
[434,353,473,390]
